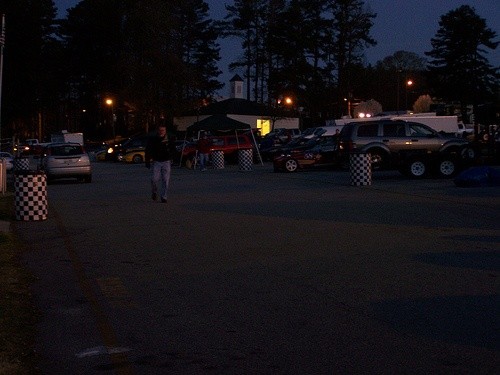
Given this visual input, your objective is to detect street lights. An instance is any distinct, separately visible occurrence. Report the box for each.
[105,98,116,138]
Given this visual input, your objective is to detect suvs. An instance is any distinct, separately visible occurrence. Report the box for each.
[334,120,469,171]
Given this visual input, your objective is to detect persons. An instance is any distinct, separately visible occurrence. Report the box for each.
[149,122,178,203]
[196,135,213,171]
[282,129,292,145]
[333,129,340,138]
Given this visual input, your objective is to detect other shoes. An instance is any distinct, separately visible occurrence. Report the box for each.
[162,196,168,203]
[152,194,157,201]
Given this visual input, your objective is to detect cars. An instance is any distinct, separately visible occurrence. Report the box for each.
[274,135,339,173]
[0,129,189,176]
[33,142,92,183]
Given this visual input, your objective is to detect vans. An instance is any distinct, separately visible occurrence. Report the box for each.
[177,133,253,172]
[261,109,500,171]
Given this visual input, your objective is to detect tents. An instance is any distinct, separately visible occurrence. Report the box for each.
[180,115,264,176]
